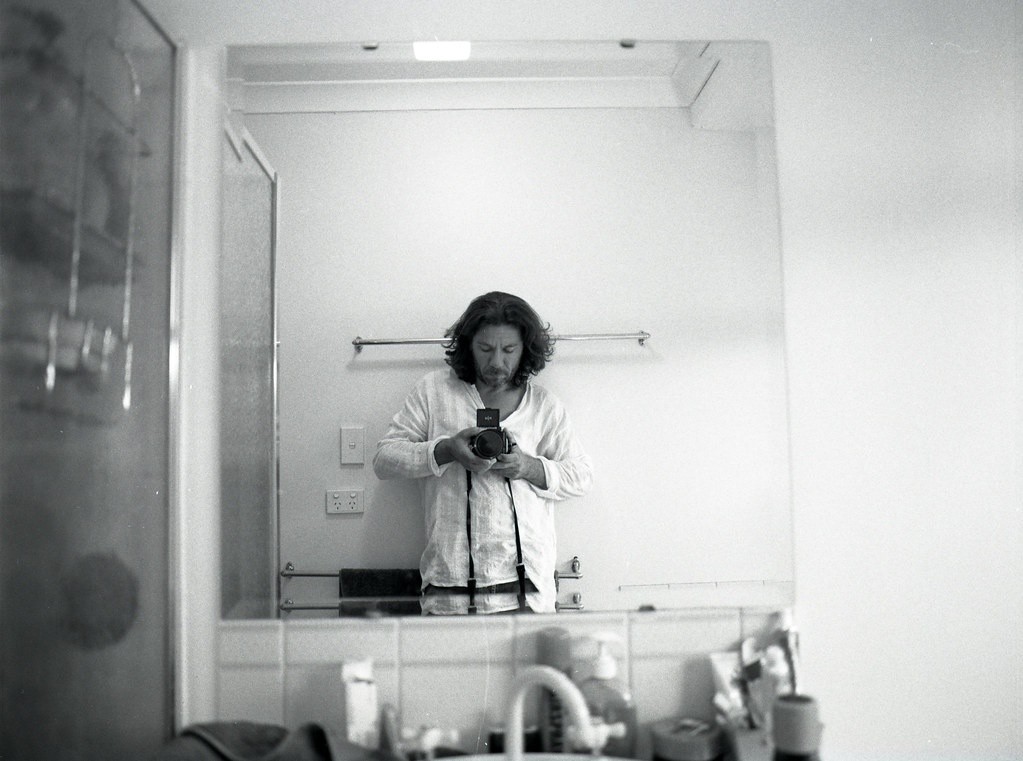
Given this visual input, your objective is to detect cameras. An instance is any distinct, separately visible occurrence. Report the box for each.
[470,407,509,459]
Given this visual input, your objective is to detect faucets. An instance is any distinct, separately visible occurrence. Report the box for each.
[506,665,625,761]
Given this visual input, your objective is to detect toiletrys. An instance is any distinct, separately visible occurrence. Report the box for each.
[707,611,800,729]
[539,627,637,758]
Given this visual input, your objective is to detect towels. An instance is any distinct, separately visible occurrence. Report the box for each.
[156,721,389,761]
[338,568,422,617]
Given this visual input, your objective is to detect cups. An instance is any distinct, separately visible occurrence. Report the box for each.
[773,694,820,757]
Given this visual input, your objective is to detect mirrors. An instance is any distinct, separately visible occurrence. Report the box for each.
[223,41,799,619]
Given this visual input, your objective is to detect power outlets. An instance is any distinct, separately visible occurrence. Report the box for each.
[326,489,366,514]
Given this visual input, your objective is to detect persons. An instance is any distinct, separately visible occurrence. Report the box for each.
[372,290,590,596]
